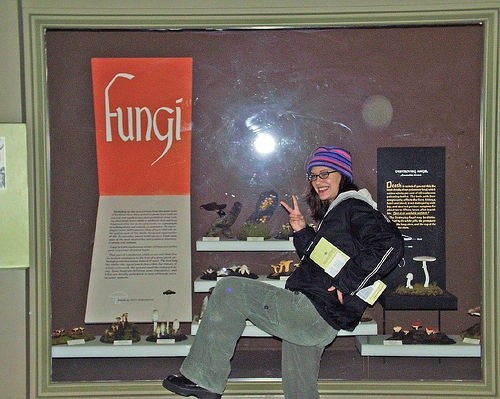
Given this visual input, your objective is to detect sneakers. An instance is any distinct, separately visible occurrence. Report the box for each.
[163,374,221,399]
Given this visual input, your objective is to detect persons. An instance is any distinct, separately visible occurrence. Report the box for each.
[161,145,405,399]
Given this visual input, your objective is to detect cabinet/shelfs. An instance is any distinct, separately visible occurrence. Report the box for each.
[51,240,482,359]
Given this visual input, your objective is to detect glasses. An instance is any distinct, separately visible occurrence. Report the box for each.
[308,171,337,181]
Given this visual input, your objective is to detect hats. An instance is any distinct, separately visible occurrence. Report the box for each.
[306,146,353,183]
